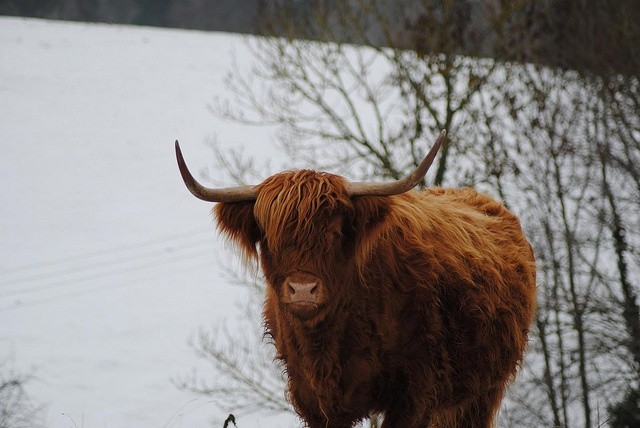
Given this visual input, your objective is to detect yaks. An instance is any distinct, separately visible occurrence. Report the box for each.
[175,128,535,426]
